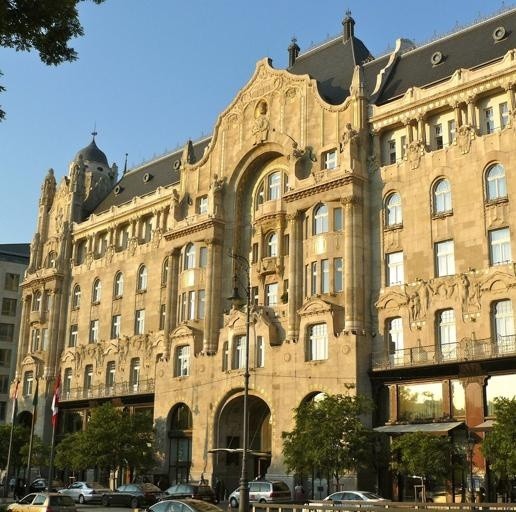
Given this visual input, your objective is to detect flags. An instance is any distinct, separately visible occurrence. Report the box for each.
[50,371,62,429]
[31,384,38,406]
[11,380,19,400]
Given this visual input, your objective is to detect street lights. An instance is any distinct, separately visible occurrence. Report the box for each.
[226,271,252,512]
[466,429,477,511]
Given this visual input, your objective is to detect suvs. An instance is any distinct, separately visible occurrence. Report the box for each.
[6,490,78,512]
[227,479,292,508]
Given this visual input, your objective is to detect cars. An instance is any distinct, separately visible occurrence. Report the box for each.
[57,480,112,503]
[144,498,226,512]
[300,489,392,512]
[100,482,170,508]
[164,482,219,504]
[27,477,66,492]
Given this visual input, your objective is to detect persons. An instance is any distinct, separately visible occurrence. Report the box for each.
[198,474,209,486]
[8,475,16,492]
[293,480,305,501]
[212,475,223,505]
